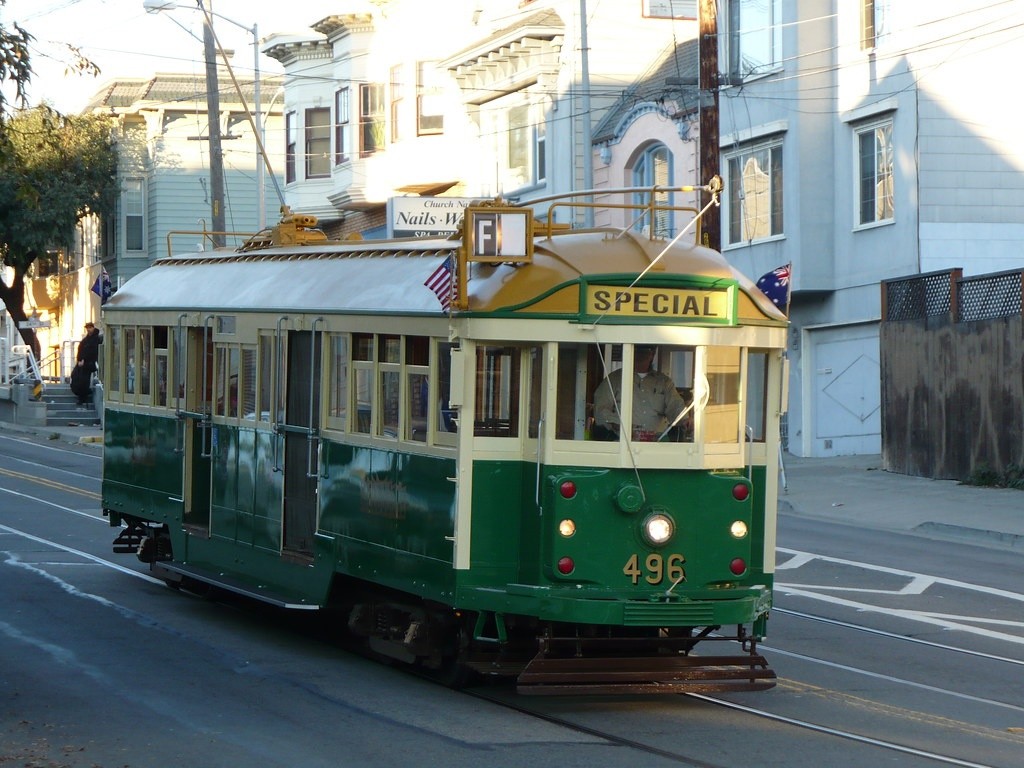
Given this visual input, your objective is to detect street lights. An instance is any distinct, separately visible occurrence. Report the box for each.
[142,0,267,234]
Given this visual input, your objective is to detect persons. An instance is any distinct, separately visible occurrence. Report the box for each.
[76,322,103,405]
[592,343,693,442]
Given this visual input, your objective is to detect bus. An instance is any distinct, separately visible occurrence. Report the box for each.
[99,0,792,699]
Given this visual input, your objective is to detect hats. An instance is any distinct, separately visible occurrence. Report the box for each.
[85,323,94,328]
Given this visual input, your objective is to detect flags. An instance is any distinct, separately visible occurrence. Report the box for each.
[424,255,458,310]
[91,267,111,299]
[756,264,790,306]
